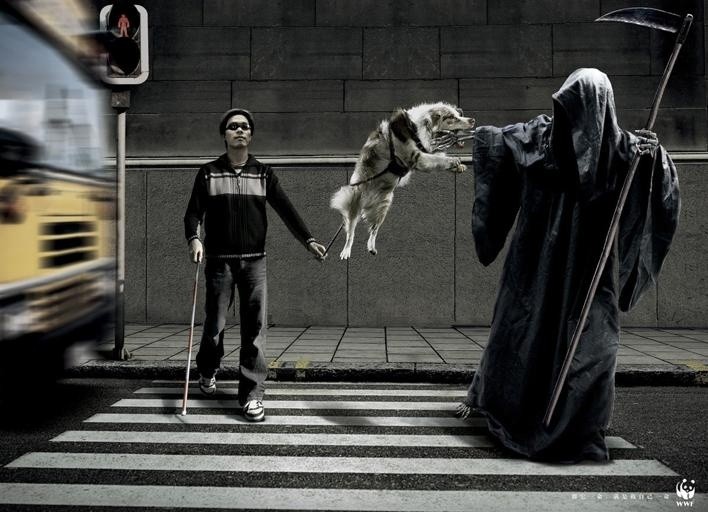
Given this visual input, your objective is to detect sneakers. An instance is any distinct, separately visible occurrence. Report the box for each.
[241,388,265,422]
[198,364,217,395]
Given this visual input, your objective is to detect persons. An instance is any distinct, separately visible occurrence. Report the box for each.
[183,108,329,421]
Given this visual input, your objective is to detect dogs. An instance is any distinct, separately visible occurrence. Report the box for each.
[329,99,476,258]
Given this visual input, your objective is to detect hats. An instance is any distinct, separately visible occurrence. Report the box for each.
[219,107,255,135]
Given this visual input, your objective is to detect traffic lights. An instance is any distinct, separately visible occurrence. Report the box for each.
[96,2,152,88]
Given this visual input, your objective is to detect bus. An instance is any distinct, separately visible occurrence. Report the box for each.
[0,0,118,387]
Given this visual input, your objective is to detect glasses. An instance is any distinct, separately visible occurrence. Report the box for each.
[226,122,251,132]
